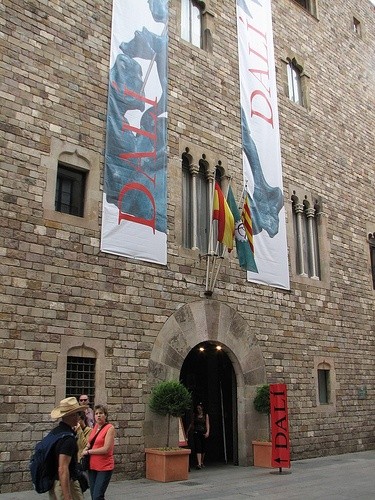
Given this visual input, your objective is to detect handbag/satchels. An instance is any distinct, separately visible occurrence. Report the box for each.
[78,452,89,471]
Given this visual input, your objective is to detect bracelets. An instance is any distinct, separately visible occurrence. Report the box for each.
[87,450,90,455]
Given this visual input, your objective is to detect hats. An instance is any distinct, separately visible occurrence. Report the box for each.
[50,396,86,418]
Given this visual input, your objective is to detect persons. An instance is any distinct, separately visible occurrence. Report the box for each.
[185,402,210,469]
[47,397,90,500]
[72,394,115,500]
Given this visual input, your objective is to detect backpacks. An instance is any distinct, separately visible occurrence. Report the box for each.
[28,431,74,493]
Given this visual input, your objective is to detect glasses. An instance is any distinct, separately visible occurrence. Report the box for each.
[79,398,87,401]
[77,411,81,417]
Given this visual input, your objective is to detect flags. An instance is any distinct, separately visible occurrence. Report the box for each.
[212,182,234,249]
[239,187,262,254]
[226,184,259,273]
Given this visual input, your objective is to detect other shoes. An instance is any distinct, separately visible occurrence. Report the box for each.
[195,465,201,469]
[201,464,208,468]
